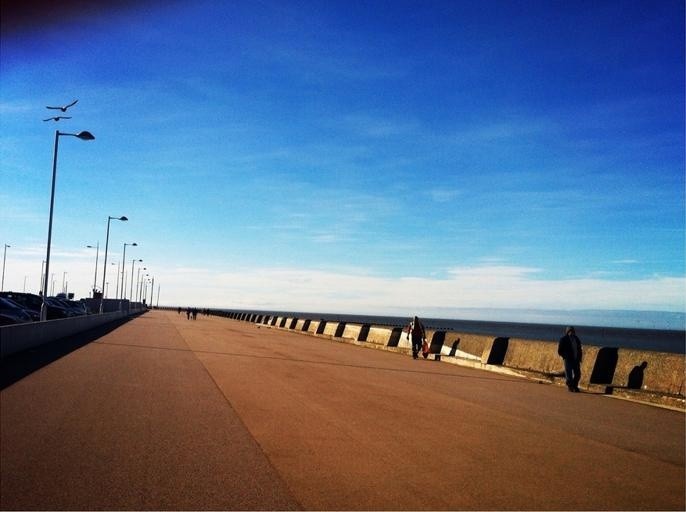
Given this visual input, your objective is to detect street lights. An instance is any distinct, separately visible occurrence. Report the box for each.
[121,269,127,300]
[111,262,119,299]
[86,241,98,292]
[102,215,128,298]
[23,261,69,298]
[105,282,109,298]
[1,243,11,291]
[130,259,151,309]
[120,242,138,299]
[40,130,94,323]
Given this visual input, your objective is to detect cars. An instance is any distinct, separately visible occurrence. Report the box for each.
[0,292,146,325]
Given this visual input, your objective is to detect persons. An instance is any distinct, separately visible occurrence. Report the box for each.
[556,326,583,393]
[175,306,211,321]
[406,315,426,359]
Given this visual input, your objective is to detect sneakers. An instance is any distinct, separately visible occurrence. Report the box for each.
[568,385,580,393]
